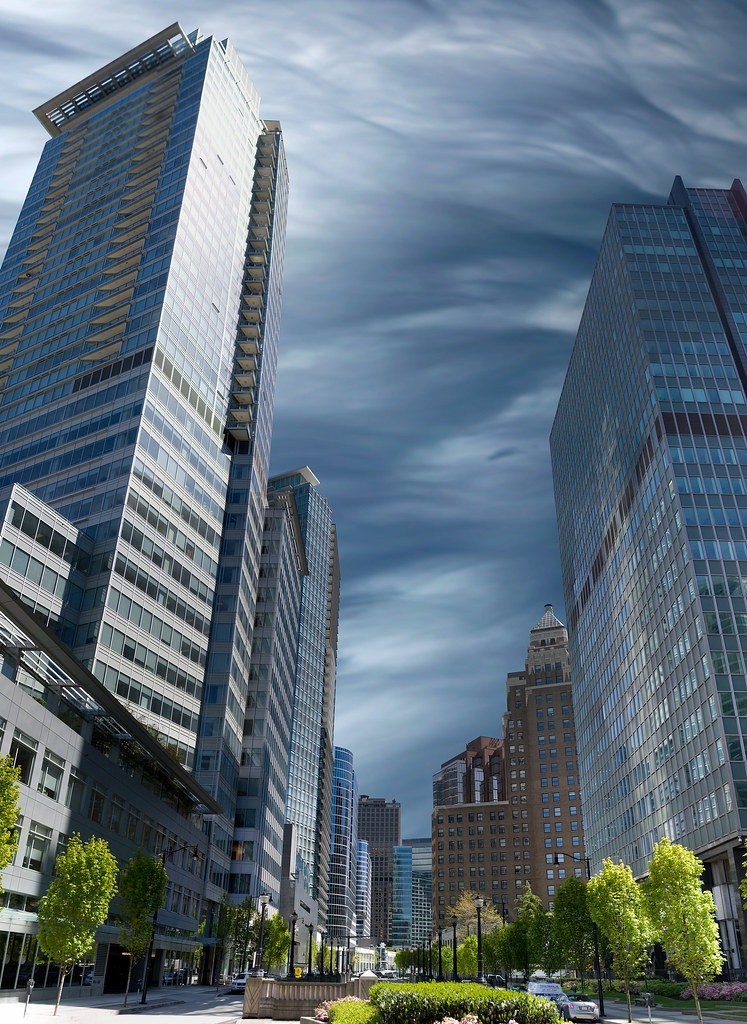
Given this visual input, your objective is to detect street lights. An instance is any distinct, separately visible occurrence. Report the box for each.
[305,924,314,976]
[319,931,325,976]
[138,845,199,1004]
[287,910,298,978]
[427,933,433,979]
[328,938,333,975]
[474,892,486,983]
[437,925,444,980]
[451,911,459,981]
[553,851,607,1018]
[250,892,270,977]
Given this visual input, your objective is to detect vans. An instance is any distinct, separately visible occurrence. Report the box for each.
[524,981,564,1002]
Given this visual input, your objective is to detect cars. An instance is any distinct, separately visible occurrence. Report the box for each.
[163,967,185,986]
[565,994,599,1024]
[231,972,252,993]
[79,963,95,985]
